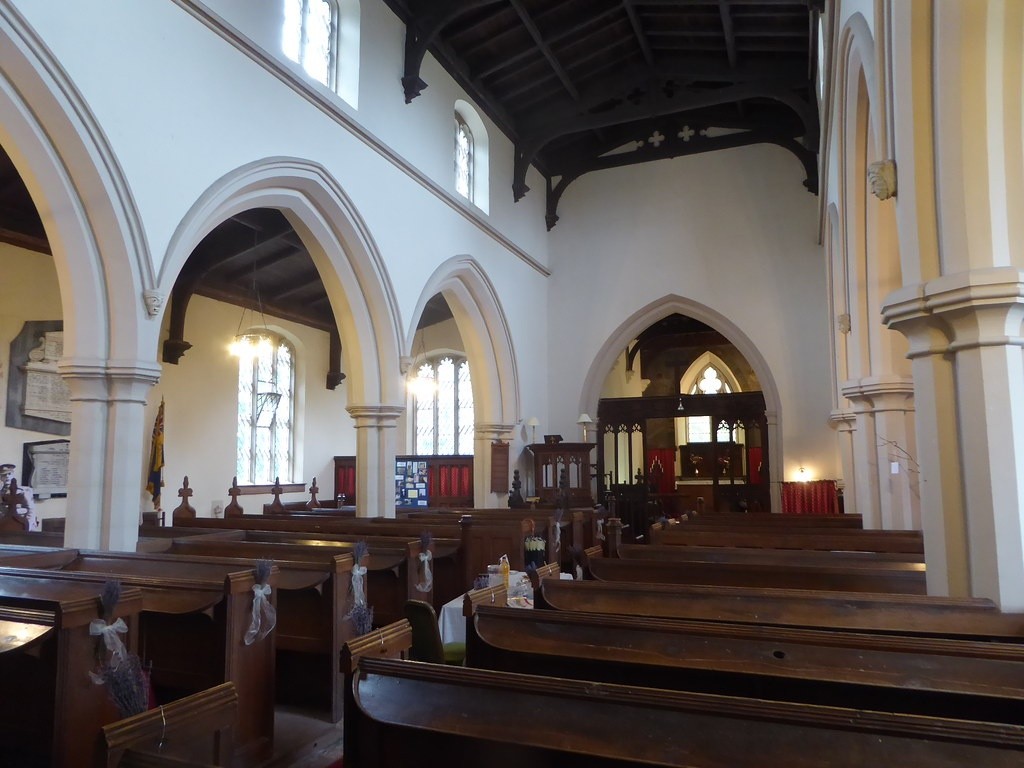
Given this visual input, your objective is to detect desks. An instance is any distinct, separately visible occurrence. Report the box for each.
[0,468,1024,768]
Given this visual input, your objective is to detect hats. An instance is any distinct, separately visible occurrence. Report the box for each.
[0,464,15,475]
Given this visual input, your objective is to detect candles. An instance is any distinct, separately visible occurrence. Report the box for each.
[649,456,664,473]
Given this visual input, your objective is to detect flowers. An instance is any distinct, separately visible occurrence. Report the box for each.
[349,603,374,635]
[474,576,489,591]
[101,578,123,623]
[420,529,432,555]
[95,650,153,718]
[254,552,274,586]
[525,535,545,567]
[352,538,369,566]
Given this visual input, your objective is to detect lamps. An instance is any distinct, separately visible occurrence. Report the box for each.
[527,418,540,443]
[577,414,593,442]
[228,231,273,360]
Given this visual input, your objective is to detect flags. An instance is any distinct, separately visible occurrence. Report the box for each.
[145,394,165,511]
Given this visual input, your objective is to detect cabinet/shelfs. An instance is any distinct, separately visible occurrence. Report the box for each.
[528,434,598,507]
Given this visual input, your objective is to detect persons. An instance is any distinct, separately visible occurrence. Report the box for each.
[0,464,40,532]
